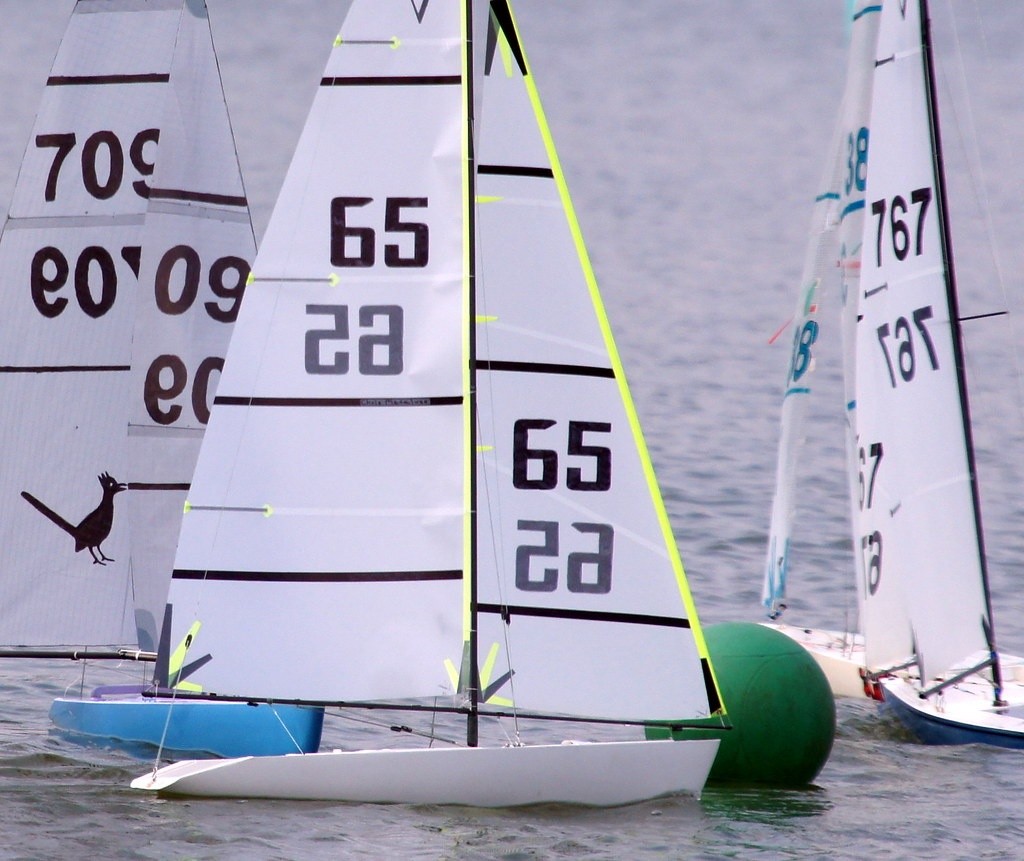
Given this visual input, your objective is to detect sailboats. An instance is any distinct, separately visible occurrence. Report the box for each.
[0,0,366,755]
[759,0,1022,761]
[128,0,745,801]
